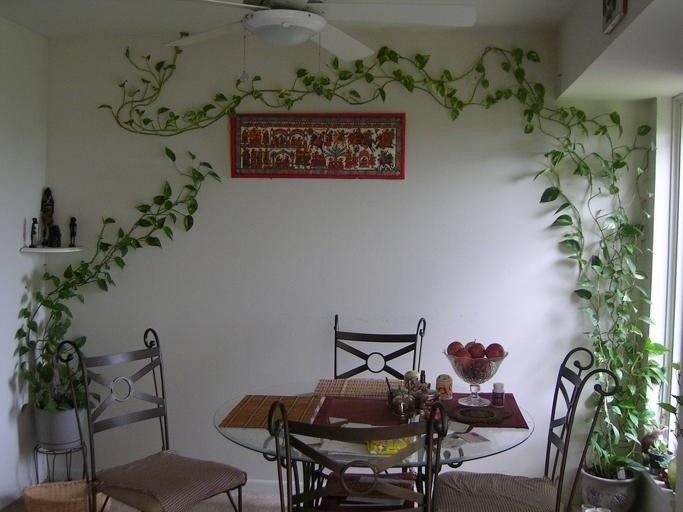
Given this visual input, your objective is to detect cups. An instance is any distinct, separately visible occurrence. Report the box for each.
[393,395,415,415]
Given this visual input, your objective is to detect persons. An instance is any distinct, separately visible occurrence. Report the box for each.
[29,217,39,248]
[39,186,56,244]
[68,216,78,245]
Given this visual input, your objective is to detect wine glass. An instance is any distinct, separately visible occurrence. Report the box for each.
[443,349,509,408]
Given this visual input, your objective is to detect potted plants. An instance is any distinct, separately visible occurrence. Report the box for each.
[11,145,218,450]
[99,43,682,512]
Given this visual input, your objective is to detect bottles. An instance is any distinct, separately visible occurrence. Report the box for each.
[420,371,426,384]
[491,382,505,408]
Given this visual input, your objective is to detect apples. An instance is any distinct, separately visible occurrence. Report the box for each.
[447,341,504,382]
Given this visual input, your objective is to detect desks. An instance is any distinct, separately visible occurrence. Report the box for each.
[212,378,535,511]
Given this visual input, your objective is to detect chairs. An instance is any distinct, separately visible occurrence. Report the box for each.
[330,309,418,511]
[54,323,248,511]
[431,345,620,511]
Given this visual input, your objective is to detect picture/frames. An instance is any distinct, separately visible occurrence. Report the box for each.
[229,110,407,180]
[602,0,627,35]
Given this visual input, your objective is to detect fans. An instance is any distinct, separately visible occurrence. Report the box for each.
[165,0,479,61]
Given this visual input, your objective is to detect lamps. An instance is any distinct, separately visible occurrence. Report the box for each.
[239,8,328,84]
